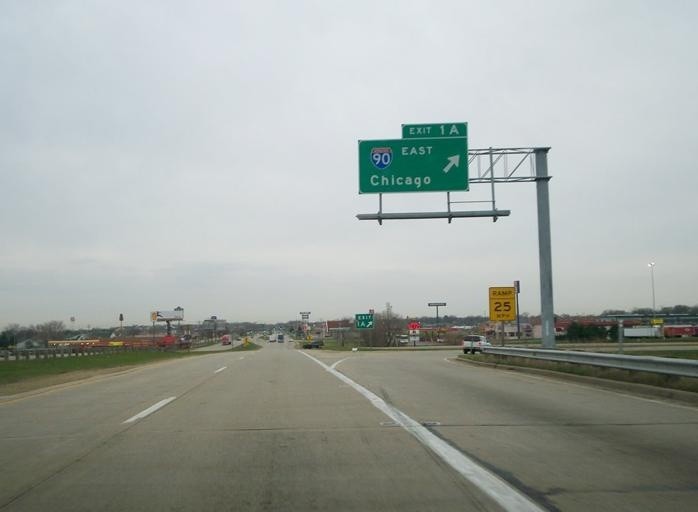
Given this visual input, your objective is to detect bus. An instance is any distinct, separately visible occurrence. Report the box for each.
[277,334,285,343]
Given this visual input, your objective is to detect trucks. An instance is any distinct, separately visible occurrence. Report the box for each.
[269,334,276,342]
[565,324,697,339]
[223,334,232,346]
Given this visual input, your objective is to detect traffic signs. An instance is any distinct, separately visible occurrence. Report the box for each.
[357,121,469,194]
[355,313,373,329]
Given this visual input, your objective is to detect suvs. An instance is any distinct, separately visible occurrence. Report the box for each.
[461,334,489,354]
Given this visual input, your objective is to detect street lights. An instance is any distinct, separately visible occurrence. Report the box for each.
[646,254,656,322]
